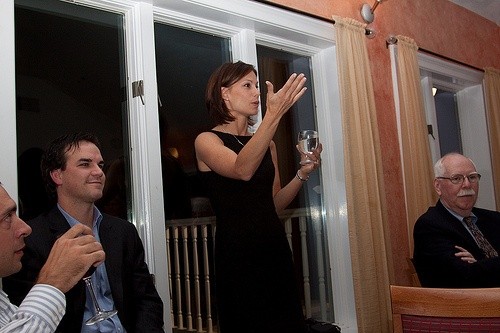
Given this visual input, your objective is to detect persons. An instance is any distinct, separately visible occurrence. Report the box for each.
[0,130,165,332]
[412,151,500,288]
[194,60,323,333]
[0,181,106,333]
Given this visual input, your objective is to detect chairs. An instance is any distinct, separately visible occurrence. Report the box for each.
[388,285,500,333]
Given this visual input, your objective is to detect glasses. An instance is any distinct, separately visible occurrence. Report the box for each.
[436,173,481,184]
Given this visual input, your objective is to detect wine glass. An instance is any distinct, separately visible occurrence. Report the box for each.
[59,235,119,326]
[298,130,319,165]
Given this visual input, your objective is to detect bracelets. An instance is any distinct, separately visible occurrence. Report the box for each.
[295,169,309,183]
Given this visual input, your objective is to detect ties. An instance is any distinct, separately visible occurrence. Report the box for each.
[463,216,499,259]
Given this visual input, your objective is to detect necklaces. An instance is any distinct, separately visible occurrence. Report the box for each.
[217,122,254,147]
